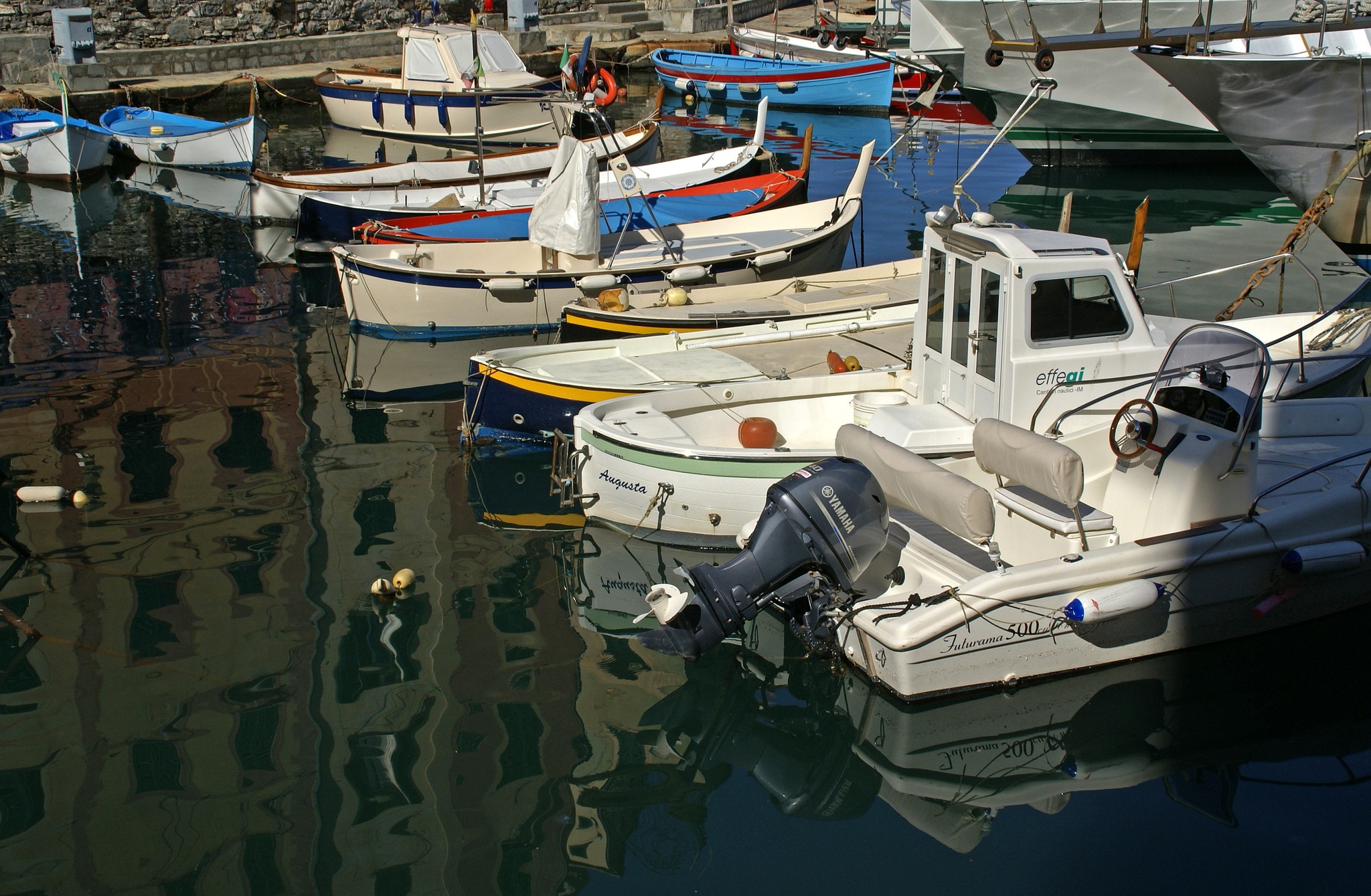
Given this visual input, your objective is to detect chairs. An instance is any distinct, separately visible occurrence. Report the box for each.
[972,417,1120,567]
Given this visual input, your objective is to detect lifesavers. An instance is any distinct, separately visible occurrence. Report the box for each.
[581,65,617,107]
[565,53,599,94]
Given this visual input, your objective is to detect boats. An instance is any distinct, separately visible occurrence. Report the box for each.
[650,47,896,112]
[725,0,1371,283]
[313,23,617,155]
[461,217,1371,705]
[246,95,879,344]
[1,167,250,281]
[337,329,557,416]
[565,529,752,641]
[0,79,268,180]
[823,609,1371,860]
[455,423,585,528]
[653,85,897,169]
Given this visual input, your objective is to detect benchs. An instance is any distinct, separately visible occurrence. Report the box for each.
[834,424,1016,580]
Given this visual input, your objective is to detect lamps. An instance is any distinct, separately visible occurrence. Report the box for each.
[933,204,959,226]
